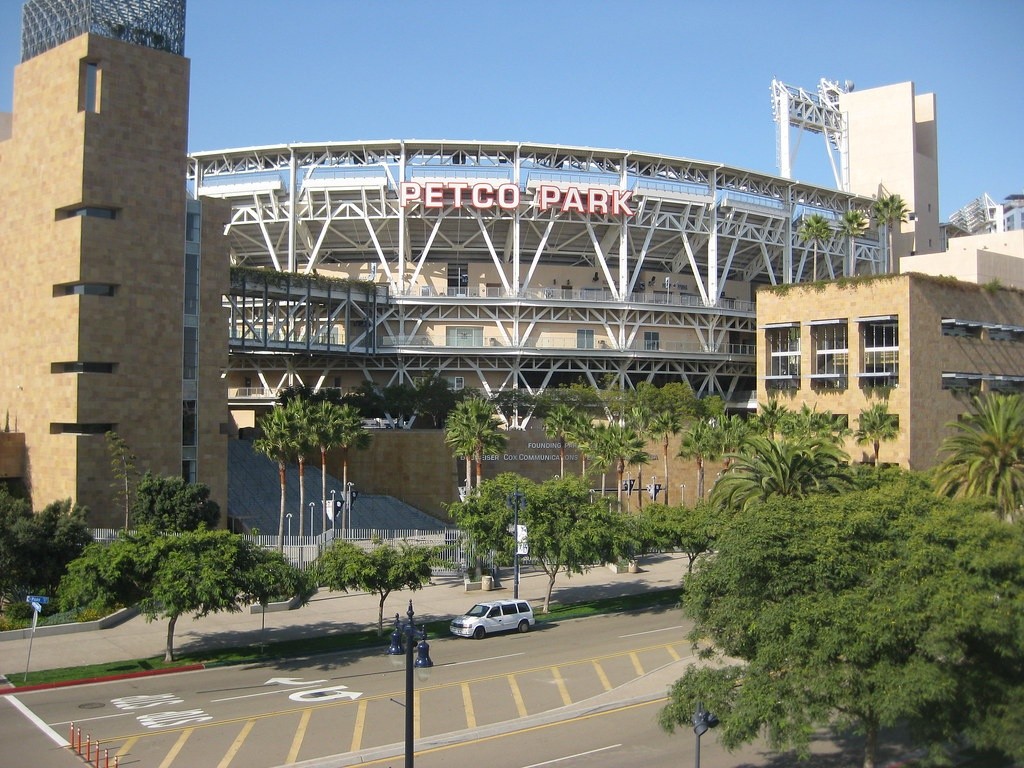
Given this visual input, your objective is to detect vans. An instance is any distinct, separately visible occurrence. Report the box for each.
[449,600,536,639]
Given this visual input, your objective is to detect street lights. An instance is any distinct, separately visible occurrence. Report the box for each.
[507,482,527,600]
[386,598,433,768]
[693,701,720,768]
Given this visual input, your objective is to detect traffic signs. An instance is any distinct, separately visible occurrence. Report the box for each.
[26,595,50,614]
